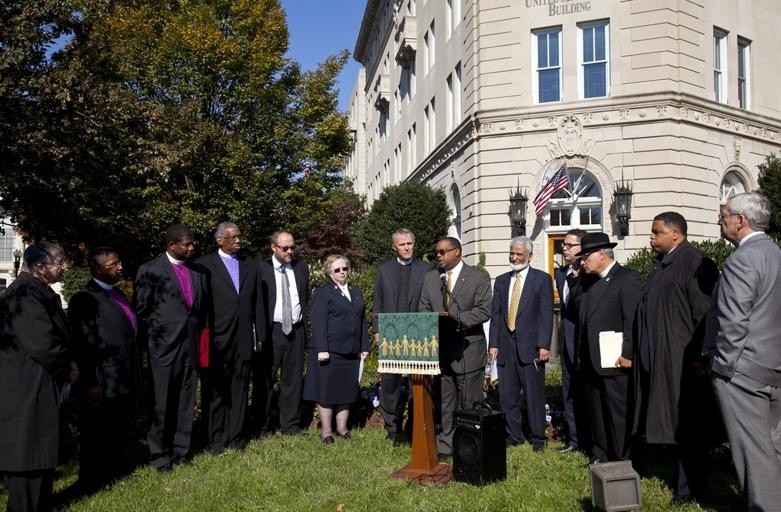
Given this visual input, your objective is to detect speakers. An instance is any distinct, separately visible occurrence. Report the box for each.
[453,401,506,486]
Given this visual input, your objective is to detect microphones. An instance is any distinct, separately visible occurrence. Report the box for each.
[440,286,460,309]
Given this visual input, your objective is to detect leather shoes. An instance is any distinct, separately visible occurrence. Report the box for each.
[336,430,350,439]
[533,442,602,467]
[324,436,333,444]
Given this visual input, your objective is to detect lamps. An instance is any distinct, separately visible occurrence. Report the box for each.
[613,178,633,236]
[508,175,529,237]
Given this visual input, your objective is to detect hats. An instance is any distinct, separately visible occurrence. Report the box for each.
[575,233,617,256]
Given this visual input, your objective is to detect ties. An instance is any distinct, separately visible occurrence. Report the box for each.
[508,273,521,332]
[280,265,293,336]
[447,272,452,304]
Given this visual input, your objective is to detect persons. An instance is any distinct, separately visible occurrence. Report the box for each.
[2,239,78,510]
[373,227,431,444]
[556,229,588,456]
[489,235,554,451]
[635,211,724,503]
[567,232,642,466]
[418,238,492,459]
[194,222,265,453]
[703,193,781,510]
[304,254,370,444]
[132,223,210,472]
[250,231,310,437]
[66,247,144,496]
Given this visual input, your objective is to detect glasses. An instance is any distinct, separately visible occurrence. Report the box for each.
[435,248,455,255]
[719,213,739,221]
[101,262,121,267]
[276,245,295,251]
[35,262,66,269]
[561,242,580,248]
[578,252,590,262]
[334,267,348,273]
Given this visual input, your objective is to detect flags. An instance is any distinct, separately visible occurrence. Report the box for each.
[532,162,569,220]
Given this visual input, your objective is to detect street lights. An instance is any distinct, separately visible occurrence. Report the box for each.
[12,249,21,281]
